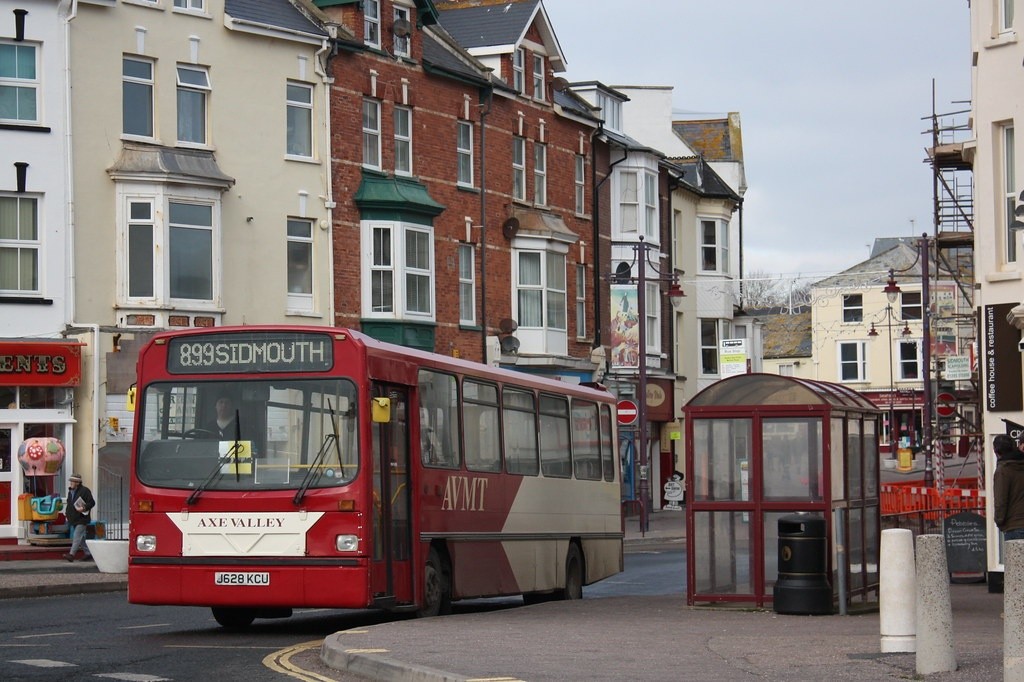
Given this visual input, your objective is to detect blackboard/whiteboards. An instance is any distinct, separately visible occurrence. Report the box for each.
[944,510,987,575]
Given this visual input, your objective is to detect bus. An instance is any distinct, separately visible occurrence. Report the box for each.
[127,323,625,629]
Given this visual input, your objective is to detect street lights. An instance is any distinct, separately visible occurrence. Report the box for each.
[604,236,687,531]
[881,233,934,489]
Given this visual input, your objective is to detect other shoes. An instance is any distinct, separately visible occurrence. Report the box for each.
[62,553,74,562]
[80,554,93,561]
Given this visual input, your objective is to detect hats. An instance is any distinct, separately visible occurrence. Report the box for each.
[68,474,82,483]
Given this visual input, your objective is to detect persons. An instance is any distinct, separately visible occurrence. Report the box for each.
[993,434,1024,619]
[63,474,96,562]
[195,392,257,462]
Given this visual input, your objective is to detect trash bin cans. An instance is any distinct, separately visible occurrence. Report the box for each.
[773,513,833,616]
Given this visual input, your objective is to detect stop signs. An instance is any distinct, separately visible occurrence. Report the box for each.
[938,392,957,417]
[617,400,638,425]
